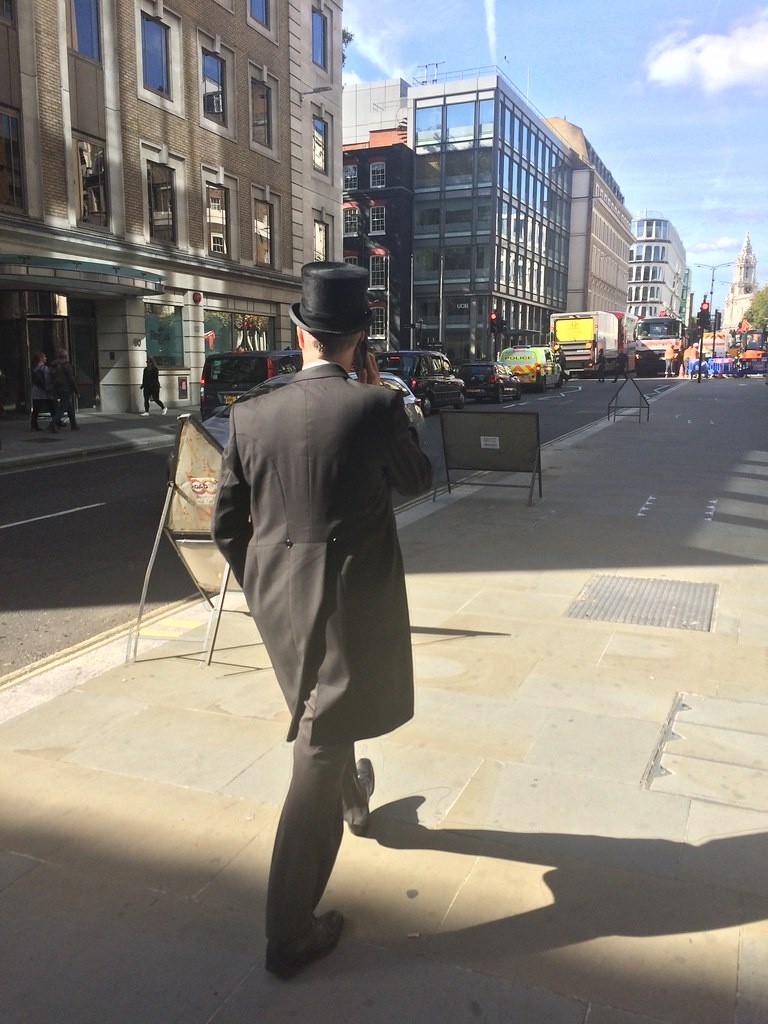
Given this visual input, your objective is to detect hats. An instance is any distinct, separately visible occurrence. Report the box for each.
[288,261,375,335]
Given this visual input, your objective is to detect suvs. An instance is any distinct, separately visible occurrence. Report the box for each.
[373,350,468,417]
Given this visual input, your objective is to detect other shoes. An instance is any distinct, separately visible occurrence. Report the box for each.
[162,407,167,415]
[57,422,66,426]
[140,412,149,416]
[71,426,81,430]
[31,424,42,430]
[49,419,58,433]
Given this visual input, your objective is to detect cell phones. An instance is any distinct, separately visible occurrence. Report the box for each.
[352,335,369,378]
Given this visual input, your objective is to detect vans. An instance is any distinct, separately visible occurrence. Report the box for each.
[497,344,565,393]
[199,348,305,424]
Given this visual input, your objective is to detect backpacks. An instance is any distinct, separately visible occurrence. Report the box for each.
[48,359,69,384]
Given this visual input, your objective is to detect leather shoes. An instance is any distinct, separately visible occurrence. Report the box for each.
[266,910,343,976]
[348,758,374,836]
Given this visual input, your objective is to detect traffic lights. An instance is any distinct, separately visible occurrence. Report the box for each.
[489,313,498,333]
[696,302,712,330]
[499,317,507,333]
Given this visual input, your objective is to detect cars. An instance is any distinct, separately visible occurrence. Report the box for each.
[456,360,522,404]
[347,370,427,452]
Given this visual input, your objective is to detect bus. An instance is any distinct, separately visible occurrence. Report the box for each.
[548,310,641,378]
[632,310,692,378]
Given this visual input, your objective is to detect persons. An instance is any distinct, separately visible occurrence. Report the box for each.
[31,352,67,430]
[611,350,627,383]
[684,343,699,377]
[213,263,434,975]
[595,349,605,382]
[665,345,675,374]
[47,351,80,432]
[140,358,167,415]
